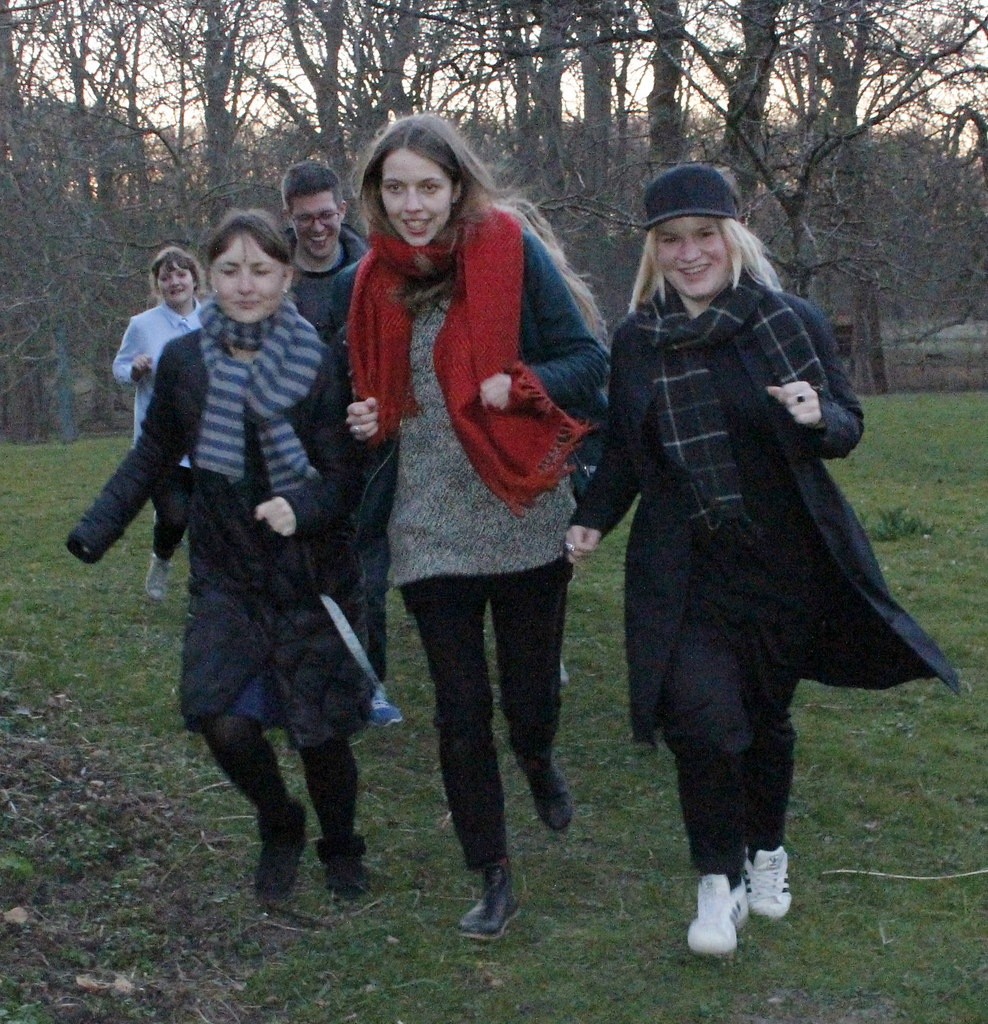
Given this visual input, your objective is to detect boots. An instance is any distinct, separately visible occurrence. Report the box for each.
[458,864,520,939]
[249,798,310,899]
[519,754,573,831]
[314,837,368,901]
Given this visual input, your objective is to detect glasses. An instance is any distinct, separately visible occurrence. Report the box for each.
[288,209,344,227]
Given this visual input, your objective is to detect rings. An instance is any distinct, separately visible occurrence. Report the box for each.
[353,424,361,434]
[565,543,574,551]
[797,393,805,402]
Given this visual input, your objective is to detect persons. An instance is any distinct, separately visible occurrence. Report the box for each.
[565,167,961,952]
[65,115,610,941]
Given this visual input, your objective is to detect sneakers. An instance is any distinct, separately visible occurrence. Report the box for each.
[145,553,170,600]
[370,684,401,730]
[741,847,791,920]
[687,872,750,954]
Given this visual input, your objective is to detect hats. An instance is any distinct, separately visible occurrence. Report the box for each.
[639,166,740,232]
